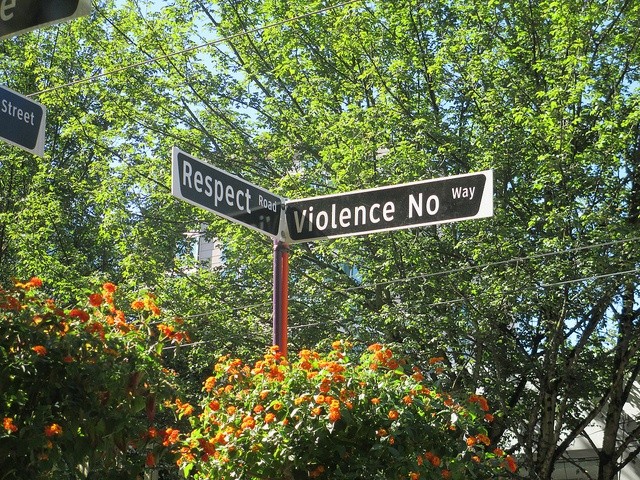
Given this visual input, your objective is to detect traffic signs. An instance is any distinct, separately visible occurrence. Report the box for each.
[284,169,493,244]
[0,85,46,159]
[0,1,91,39]
[171,145,283,238]
